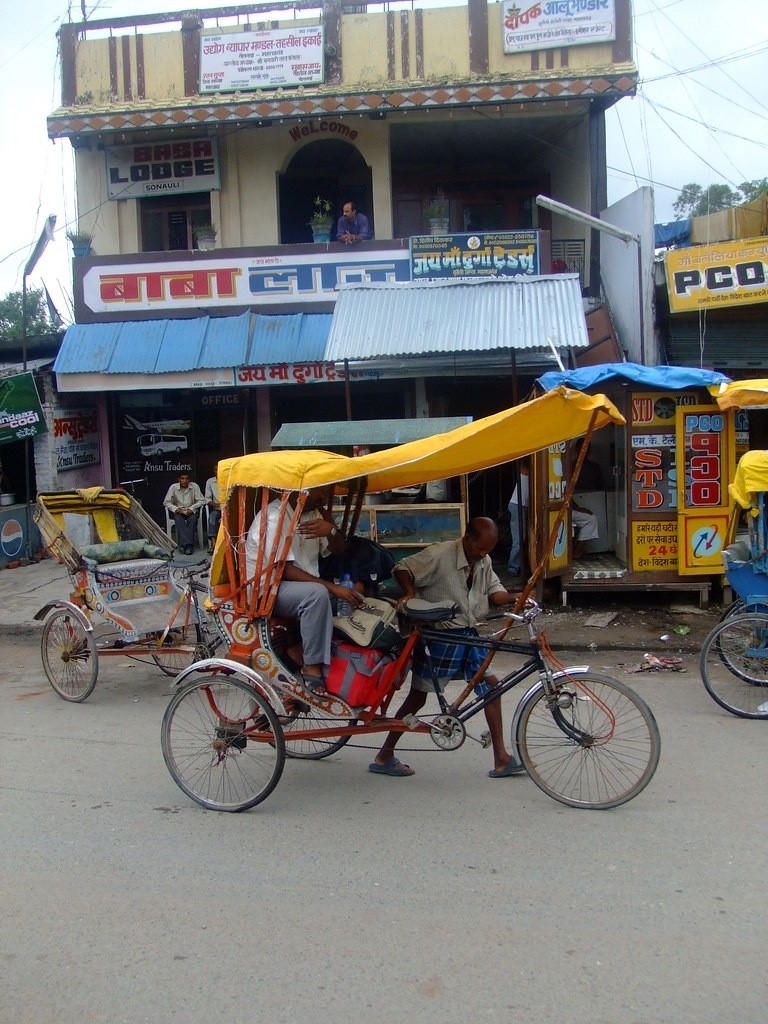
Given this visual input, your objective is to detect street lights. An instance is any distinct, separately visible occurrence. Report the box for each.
[22,215,57,372]
[536,195,645,366]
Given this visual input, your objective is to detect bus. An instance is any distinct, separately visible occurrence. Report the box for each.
[137,434,187,456]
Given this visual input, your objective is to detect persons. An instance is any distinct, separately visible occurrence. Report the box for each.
[562,437,599,561]
[336,202,372,245]
[163,470,205,555]
[205,465,221,553]
[506,455,529,577]
[369,517,536,778]
[246,485,395,702]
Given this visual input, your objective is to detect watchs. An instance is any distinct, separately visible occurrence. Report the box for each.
[329,525,337,538]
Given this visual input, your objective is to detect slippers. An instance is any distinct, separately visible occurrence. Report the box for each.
[369,757,415,776]
[489,755,539,777]
[296,670,329,702]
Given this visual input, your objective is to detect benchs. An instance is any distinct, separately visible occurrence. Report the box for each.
[77,538,170,583]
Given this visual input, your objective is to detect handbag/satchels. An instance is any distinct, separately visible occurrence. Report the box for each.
[333,597,401,649]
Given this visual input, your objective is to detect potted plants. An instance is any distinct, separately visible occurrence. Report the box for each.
[64,228,95,248]
[191,221,221,240]
[422,190,449,230]
[306,195,336,234]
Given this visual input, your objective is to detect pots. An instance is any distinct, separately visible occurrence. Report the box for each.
[0,493,15,505]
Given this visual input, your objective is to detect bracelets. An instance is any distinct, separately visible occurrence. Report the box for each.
[353,234,355,240]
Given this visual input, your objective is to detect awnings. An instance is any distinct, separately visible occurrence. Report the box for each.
[52,272,590,392]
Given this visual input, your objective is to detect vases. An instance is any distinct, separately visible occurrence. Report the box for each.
[71,247,92,257]
[312,233,331,243]
[196,239,216,249]
[429,227,450,235]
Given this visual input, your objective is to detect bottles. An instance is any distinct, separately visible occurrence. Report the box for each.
[337,574,353,619]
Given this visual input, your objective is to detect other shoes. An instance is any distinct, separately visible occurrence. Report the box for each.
[207,547,213,553]
[179,547,193,554]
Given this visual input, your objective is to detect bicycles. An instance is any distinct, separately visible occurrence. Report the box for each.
[161,384,661,813]
[28,486,225,703]
[699,450,768,720]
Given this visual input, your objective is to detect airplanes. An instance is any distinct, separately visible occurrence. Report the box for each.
[123,415,191,434]
